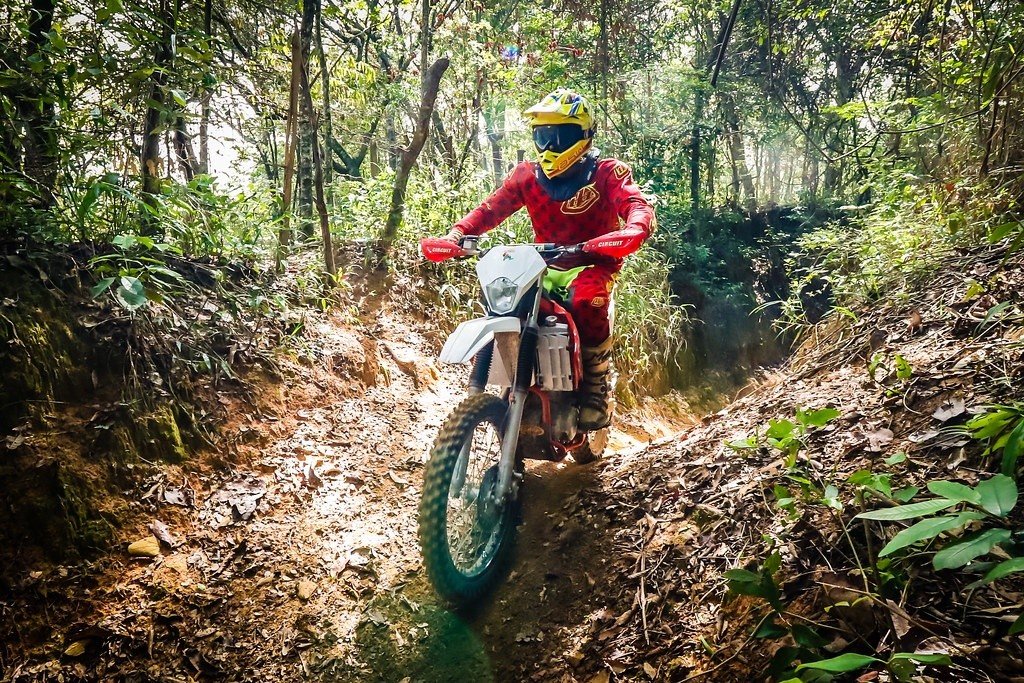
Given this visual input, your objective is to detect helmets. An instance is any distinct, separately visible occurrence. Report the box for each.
[523,89,597,180]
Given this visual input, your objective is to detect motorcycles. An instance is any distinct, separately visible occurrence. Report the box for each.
[411,234,626,618]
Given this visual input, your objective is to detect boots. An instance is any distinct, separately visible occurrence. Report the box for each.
[578,333,612,430]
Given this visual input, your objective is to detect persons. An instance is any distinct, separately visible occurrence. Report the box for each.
[434,90,657,430]
